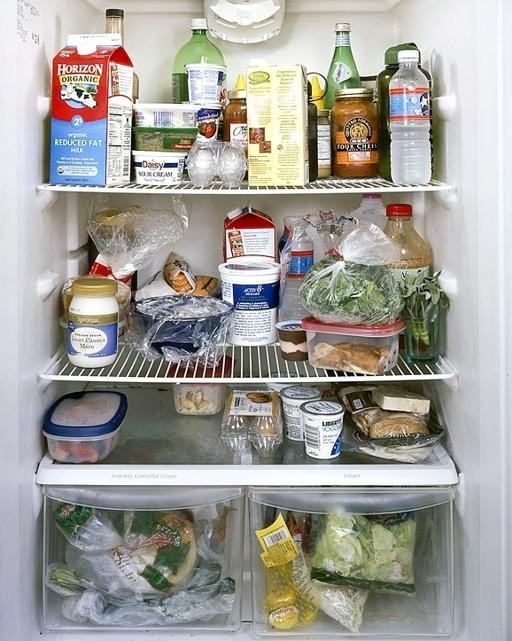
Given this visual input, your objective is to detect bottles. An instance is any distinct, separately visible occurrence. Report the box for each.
[235,73,246,90]
[325,22,360,110]
[170,17,229,105]
[281,216,316,322]
[345,193,432,289]
[387,49,432,188]
[310,75,326,110]
[375,41,430,183]
[65,278,120,369]
[104,7,141,104]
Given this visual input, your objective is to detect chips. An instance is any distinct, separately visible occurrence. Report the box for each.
[314,515,414,584]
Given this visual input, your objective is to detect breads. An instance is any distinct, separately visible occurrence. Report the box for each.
[357,408,430,435]
[319,342,391,370]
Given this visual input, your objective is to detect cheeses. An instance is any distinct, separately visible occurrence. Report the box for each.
[371,387,431,414]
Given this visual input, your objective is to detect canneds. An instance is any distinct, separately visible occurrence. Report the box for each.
[222,90,248,180]
[314,109,332,178]
[332,88,380,178]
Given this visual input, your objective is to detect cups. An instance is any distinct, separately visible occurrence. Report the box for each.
[184,61,222,106]
[196,106,221,142]
[276,317,306,363]
[220,261,280,348]
[265,375,349,462]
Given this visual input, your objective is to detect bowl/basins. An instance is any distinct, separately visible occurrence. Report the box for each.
[132,297,233,355]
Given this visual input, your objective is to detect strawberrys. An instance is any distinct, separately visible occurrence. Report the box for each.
[205,122,216,138]
[198,122,207,136]
